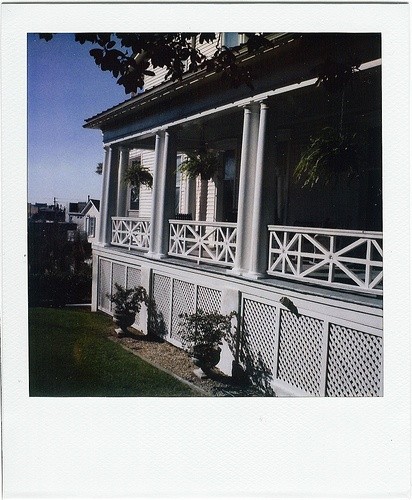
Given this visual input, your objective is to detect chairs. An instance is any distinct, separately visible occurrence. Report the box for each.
[175,213,194,236]
[292,220,366,258]
[224,207,237,223]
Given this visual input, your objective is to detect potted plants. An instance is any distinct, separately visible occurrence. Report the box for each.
[173,149,222,182]
[122,164,153,187]
[296,131,338,188]
[180,308,231,374]
[106,282,150,340]
